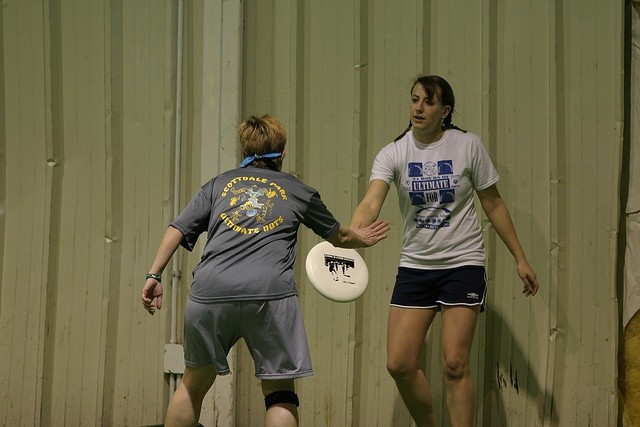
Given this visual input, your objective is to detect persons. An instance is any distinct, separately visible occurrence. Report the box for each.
[142,114,392,427]
[348,74,539,427]
[328,261,339,281]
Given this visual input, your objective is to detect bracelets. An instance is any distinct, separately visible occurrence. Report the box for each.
[145,273,162,283]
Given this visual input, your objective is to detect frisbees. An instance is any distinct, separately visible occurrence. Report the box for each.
[305,241,369,303]
